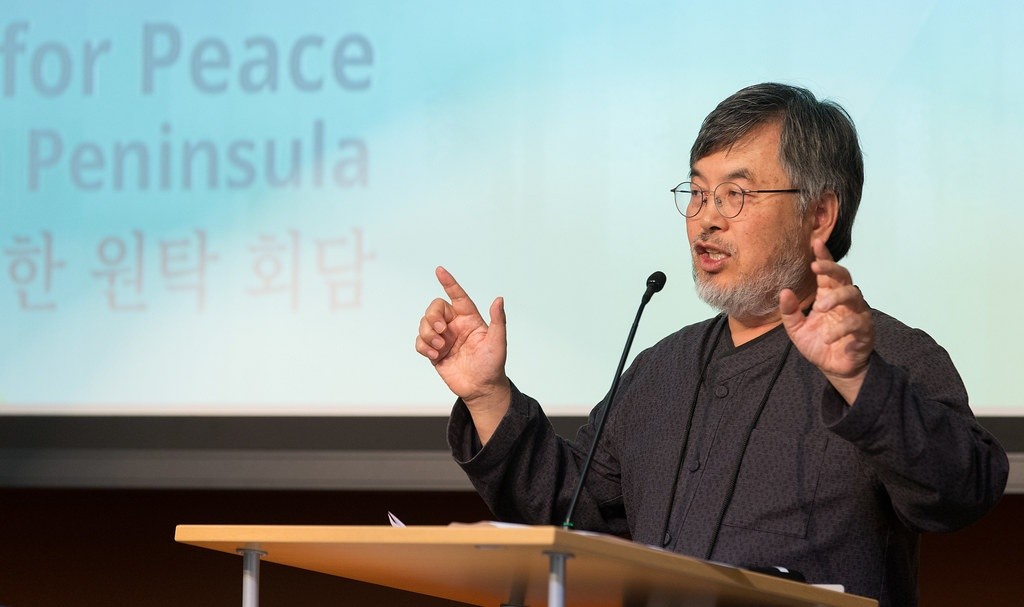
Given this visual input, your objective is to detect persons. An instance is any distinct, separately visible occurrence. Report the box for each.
[414,83,1008,607]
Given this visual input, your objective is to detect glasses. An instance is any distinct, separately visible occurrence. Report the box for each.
[670,182,810,218]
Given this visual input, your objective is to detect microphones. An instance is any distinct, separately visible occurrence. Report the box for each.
[563,270,668,529]
